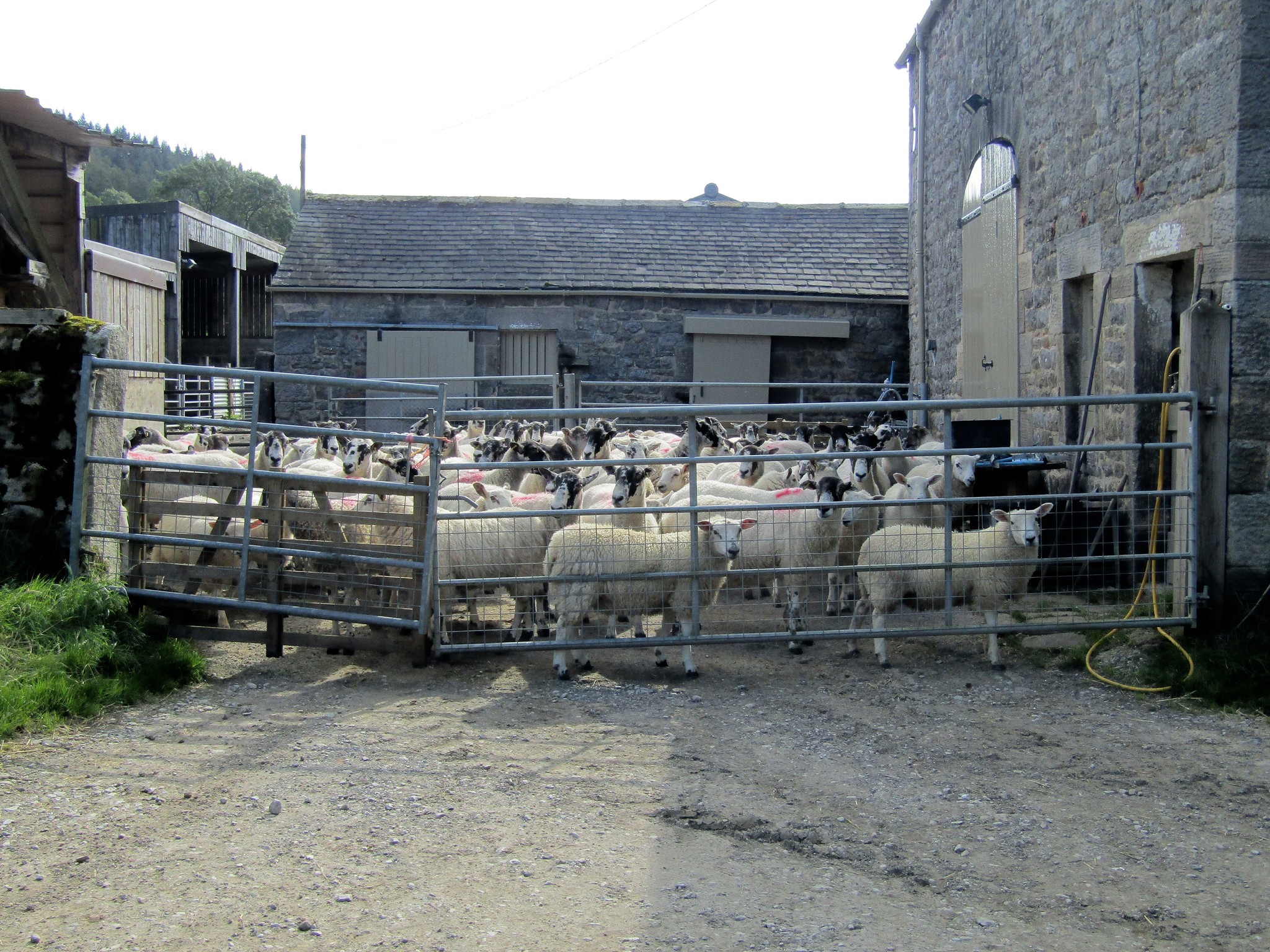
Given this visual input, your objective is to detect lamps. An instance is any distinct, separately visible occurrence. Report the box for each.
[181,257,198,270]
[961,93,992,116]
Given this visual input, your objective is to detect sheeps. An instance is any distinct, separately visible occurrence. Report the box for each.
[118,405,1054,683]
